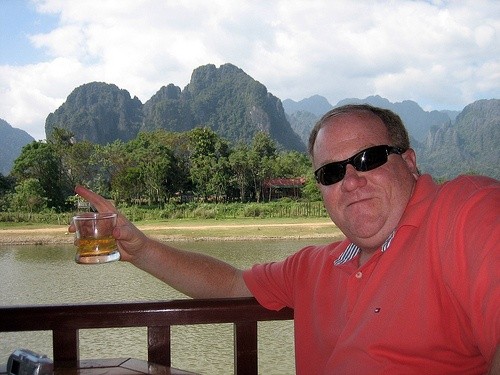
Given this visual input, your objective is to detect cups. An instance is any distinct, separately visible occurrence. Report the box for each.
[73,213,120,264]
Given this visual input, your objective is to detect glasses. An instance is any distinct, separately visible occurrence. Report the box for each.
[314,144,407,186]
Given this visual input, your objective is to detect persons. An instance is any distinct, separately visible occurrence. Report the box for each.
[69,105,500,374]
